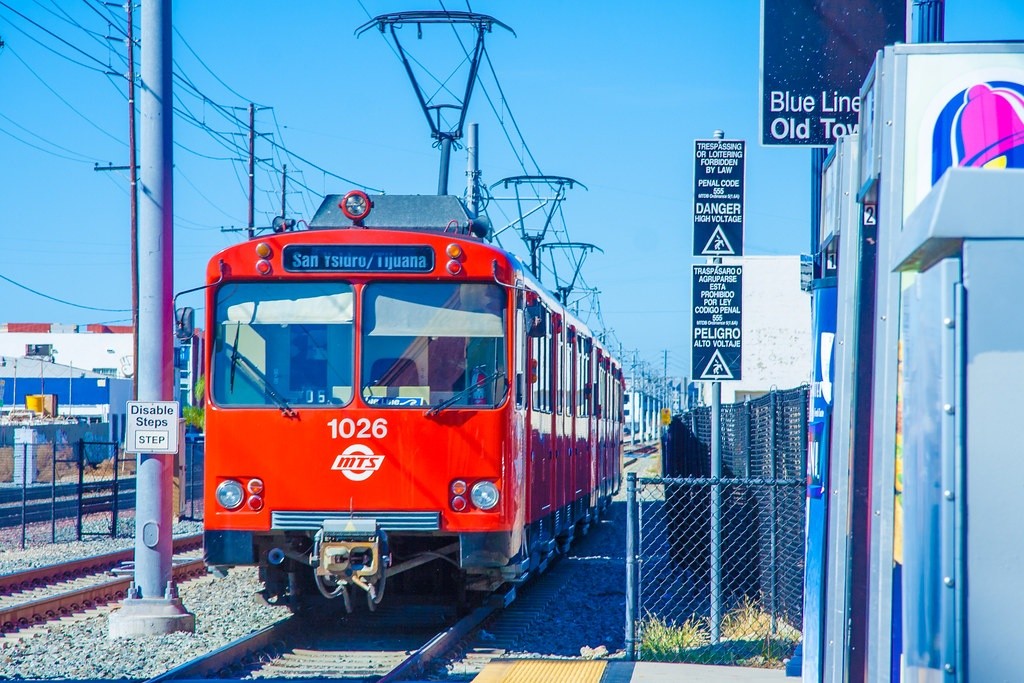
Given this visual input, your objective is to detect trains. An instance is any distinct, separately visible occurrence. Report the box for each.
[202,191,625,613]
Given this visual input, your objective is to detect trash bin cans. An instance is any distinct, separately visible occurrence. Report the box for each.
[185,433,204,501]
